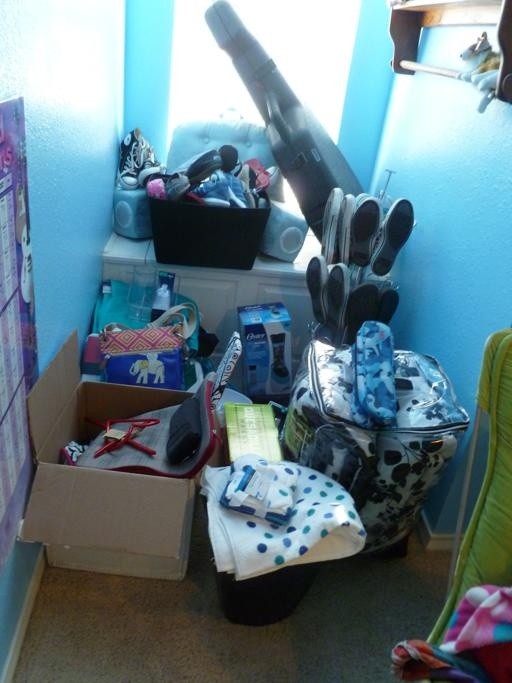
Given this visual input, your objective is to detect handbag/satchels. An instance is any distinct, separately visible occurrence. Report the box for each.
[167,398,201,465]
[82,279,199,390]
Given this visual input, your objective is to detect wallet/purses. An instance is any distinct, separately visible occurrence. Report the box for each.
[351,320,397,428]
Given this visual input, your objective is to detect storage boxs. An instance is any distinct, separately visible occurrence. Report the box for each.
[147,186,271,270]
[19,329,222,583]
[237,301,293,397]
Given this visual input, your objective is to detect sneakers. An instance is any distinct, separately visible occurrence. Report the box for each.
[146,146,281,209]
[306,188,414,348]
[117,128,161,191]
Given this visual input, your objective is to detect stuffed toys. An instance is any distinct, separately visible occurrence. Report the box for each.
[456,31,502,92]
